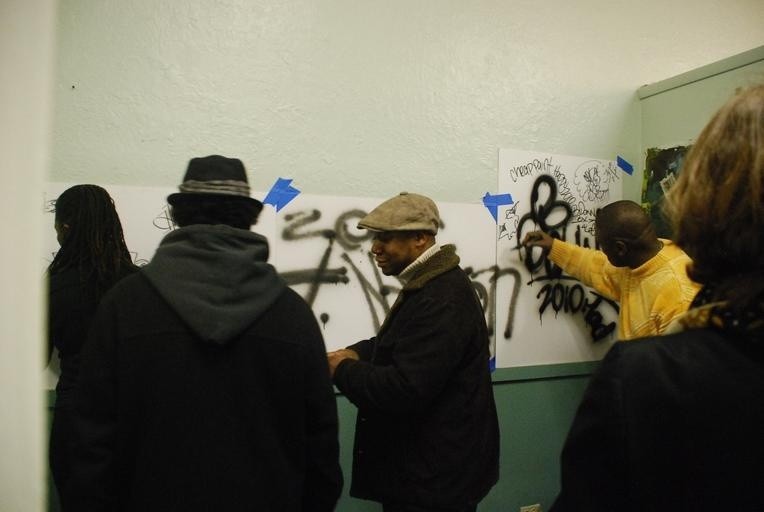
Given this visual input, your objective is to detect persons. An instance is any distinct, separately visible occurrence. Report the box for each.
[46,184,141,385]
[518,199,705,341]
[523,83,764,512]
[324,192,502,510]
[46,153,343,510]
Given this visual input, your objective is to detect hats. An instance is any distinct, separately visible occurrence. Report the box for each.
[166,154,263,215]
[357,191,441,236]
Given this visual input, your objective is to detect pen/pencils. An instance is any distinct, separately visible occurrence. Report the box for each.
[513,235,543,250]
